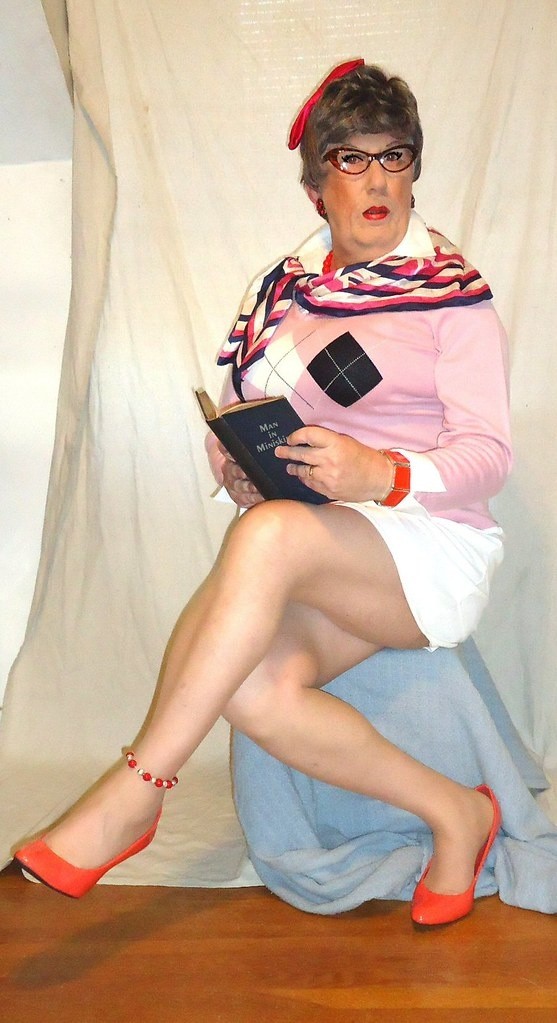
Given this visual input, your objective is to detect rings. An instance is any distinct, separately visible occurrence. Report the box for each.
[309,465,317,475]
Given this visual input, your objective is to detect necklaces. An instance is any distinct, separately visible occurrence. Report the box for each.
[322,250,333,276]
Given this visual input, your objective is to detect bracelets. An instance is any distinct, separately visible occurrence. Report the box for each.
[374,449,411,508]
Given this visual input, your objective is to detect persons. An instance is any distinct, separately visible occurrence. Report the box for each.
[13,59,515,926]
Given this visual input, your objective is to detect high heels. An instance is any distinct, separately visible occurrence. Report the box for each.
[13,797,162,899]
[410,784,501,926]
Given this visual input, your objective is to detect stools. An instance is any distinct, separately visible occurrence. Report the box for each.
[226,637,557,916]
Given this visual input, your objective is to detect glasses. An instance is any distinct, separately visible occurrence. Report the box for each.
[321,144,418,175]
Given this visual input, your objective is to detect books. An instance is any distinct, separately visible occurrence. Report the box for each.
[195,390,339,504]
